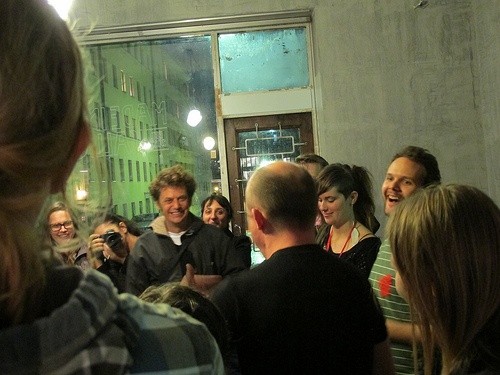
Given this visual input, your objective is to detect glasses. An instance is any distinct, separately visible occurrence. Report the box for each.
[48,220,75,232]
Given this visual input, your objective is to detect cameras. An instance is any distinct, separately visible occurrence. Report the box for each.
[100,230,123,252]
